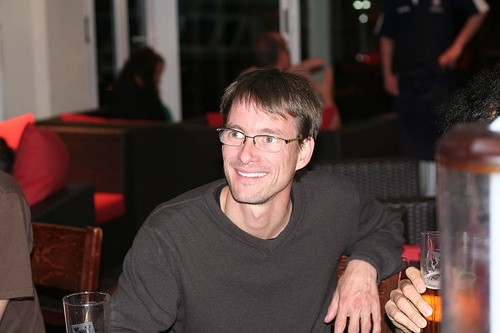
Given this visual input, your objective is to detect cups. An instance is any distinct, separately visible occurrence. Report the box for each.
[62,291,111,333]
[419,231,442,333]
[436,122,499,332]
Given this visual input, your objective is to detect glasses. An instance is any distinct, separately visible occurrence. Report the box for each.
[214,127,307,154]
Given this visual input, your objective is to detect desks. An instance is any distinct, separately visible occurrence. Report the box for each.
[10,107,206,236]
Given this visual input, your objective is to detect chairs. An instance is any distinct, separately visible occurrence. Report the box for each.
[24,81,442,333]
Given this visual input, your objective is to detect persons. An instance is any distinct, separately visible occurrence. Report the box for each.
[106,67,406,333]
[384,66,500,333]
[0,170,46,333]
[104,47,168,122]
[209,32,340,132]
[373,0,491,159]
